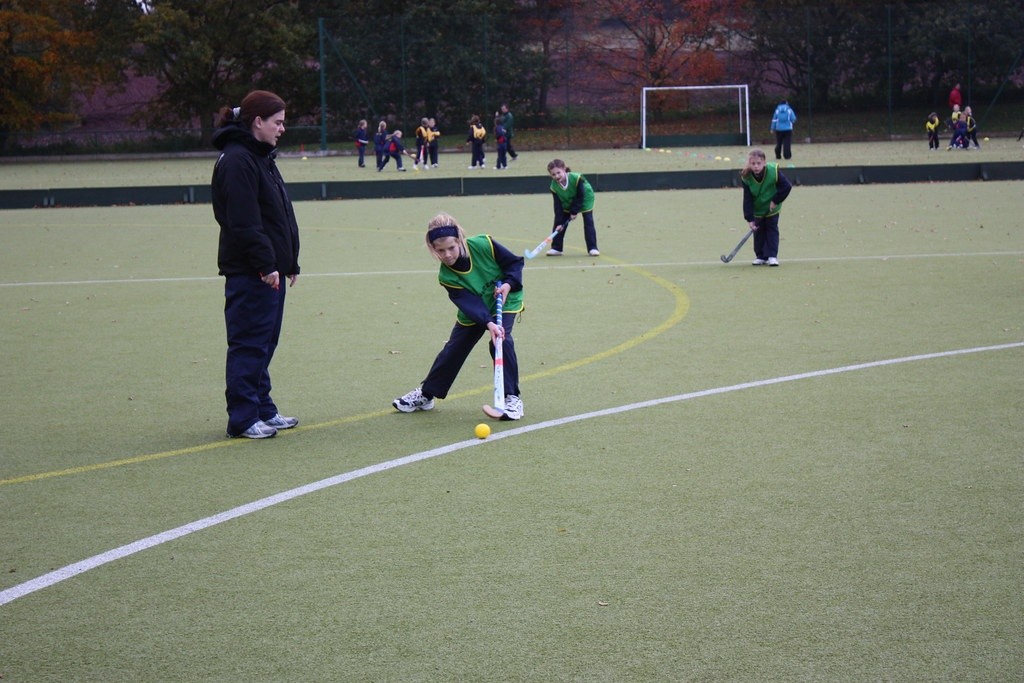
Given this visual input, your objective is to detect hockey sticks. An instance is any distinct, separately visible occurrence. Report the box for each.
[721,207,770,263]
[482,281,506,419]
[525,218,571,259]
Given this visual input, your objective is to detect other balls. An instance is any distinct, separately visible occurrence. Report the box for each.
[475,423,491,439]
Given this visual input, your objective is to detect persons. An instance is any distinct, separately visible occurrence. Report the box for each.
[769,99,796,160]
[948,82,962,128]
[392,213,525,420]
[465,115,486,169]
[378,130,408,172]
[546,159,600,256]
[946,104,979,151]
[348,119,370,167]
[374,120,387,167]
[926,112,942,151]
[492,119,508,170]
[412,117,440,171]
[210,90,301,438]
[739,149,792,266]
[495,104,518,160]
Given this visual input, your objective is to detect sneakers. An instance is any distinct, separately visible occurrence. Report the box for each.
[393,387,435,413]
[265,413,299,429]
[499,395,524,421]
[226,420,278,439]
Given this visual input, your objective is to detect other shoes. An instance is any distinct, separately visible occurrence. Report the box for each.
[589,249,600,256]
[752,258,766,265]
[546,249,563,256]
[928,143,979,153]
[768,256,779,267]
[357,154,518,170]
